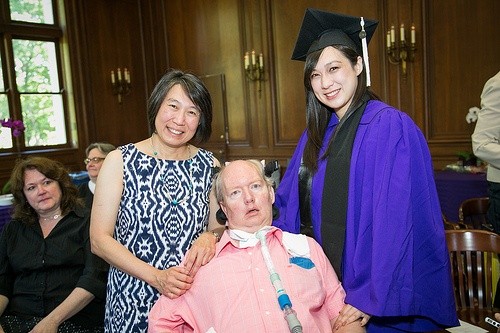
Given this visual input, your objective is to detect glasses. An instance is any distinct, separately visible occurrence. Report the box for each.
[83,156,105,164]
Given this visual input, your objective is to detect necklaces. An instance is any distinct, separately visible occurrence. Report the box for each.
[151,137,192,204]
[40,215,61,222]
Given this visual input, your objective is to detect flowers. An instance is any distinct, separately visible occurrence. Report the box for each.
[1,117,26,136]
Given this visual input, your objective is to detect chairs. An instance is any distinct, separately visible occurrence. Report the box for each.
[437,196,500,333]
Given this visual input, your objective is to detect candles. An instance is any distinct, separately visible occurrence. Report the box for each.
[411,25,415,44]
[251,50,255,66]
[112,67,131,84]
[400,24,405,40]
[387,31,391,48]
[391,25,396,43]
[259,52,264,68]
[244,52,249,70]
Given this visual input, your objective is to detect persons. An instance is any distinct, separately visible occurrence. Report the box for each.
[0,157,110,333]
[147,160,366,333]
[90,70,225,333]
[471,70,500,308]
[271,8,461,333]
[77,143,115,209]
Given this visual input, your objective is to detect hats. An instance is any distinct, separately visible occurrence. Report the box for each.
[291,7,379,86]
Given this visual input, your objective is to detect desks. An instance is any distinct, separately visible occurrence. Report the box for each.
[431,171,490,228]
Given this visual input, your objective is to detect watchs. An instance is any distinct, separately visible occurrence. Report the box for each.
[212,232,219,241]
